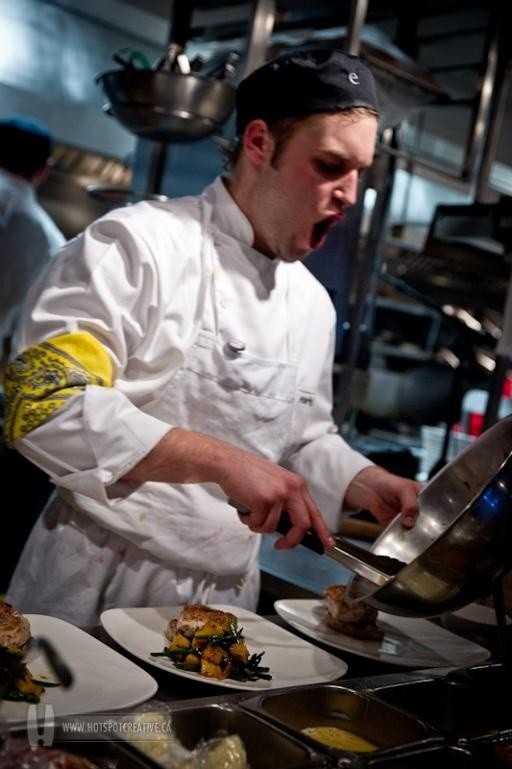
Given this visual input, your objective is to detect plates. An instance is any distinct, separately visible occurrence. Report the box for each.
[1,612,159,726]
[99,602,350,692]
[275,597,493,669]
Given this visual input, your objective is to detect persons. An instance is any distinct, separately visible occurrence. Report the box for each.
[1,114,68,353]
[2,48,422,629]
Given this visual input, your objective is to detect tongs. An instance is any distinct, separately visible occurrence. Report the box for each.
[225,495,408,587]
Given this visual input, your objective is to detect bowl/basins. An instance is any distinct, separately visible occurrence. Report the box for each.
[95,70,238,142]
[349,412,512,615]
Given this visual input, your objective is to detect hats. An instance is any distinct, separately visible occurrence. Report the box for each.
[0,114,55,162]
[234,44,383,139]
